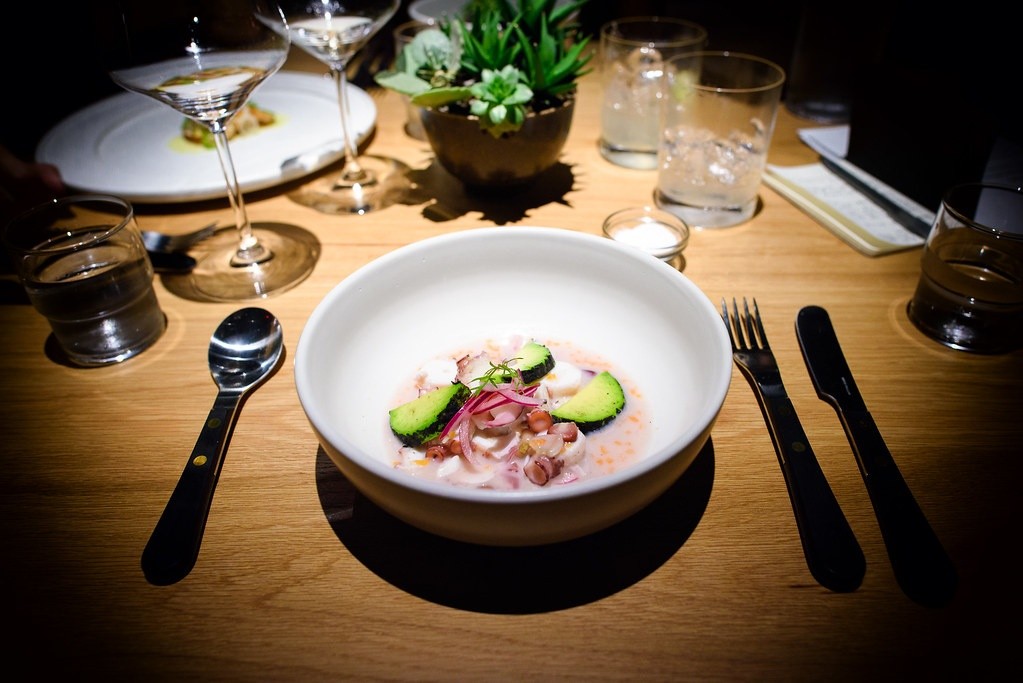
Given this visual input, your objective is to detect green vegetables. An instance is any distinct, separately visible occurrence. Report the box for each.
[466,356,533,396]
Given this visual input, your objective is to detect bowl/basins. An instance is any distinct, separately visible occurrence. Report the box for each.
[603,206,690,262]
[294,225,733,546]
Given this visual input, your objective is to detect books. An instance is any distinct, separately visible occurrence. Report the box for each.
[758,159,927,259]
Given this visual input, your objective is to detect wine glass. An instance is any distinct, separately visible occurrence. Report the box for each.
[255,2,414,216]
[92,0,322,299]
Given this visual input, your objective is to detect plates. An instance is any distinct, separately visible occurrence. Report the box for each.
[35,70,375,203]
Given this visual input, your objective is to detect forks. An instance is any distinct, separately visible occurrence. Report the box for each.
[722,297,866,593]
[140,221,219,252]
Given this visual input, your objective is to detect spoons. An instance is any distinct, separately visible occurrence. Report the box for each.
[142,308,284,585]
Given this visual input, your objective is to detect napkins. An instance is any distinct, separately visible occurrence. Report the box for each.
[758,155,938,259]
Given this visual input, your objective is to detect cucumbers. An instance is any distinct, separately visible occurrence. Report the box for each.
[550,370,626,433]
[388,381,472,446]
[499,342,555,383]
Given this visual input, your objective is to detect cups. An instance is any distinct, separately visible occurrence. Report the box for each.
[393,21,492,140]
[9,196,167,368]
[655,51,785,228]
[598,17,707,171]
[908,184,1023,353]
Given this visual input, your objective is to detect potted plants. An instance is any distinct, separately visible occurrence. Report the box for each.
[373,0,599,187]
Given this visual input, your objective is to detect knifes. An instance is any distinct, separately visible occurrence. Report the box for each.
[795,305,958,608]
[819,154,933,240]
